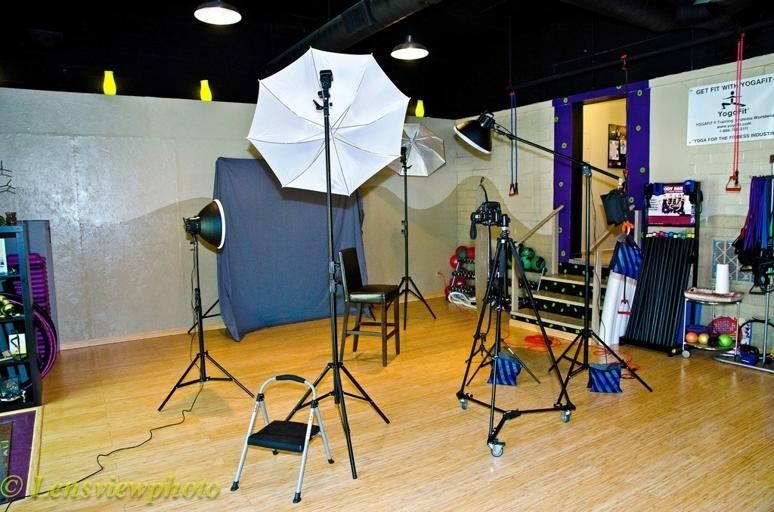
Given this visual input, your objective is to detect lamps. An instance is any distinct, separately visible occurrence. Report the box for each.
[452,111,654,405]
[193,0,243,27]
[391,19,430,61]
[157,199,254,412]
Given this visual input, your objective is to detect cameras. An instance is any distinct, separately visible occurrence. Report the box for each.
[470,200,502,226]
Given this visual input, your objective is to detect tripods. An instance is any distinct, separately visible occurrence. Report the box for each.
[466,225,541,387]
[157,234,257,412]
[281,69,390,480]
[393,162,437,331]
[494,129,653,409]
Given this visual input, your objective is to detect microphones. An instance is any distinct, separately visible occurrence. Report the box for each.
[399,146,407,162]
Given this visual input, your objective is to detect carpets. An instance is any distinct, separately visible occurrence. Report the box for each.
[0,404,42,505]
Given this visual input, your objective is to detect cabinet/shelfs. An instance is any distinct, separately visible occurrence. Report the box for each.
[0,224,44,412]
[680,290,743,359]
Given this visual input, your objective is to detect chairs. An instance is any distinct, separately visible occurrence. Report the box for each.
[340,247,400,367]
[228,375,334,504]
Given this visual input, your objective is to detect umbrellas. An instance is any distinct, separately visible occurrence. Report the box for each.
[385,121,446,179]
[246,44,412,200]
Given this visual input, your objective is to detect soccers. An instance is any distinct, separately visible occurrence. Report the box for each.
[494,242,545,272]
[444,245,475,297]
[682,329,731,347]
